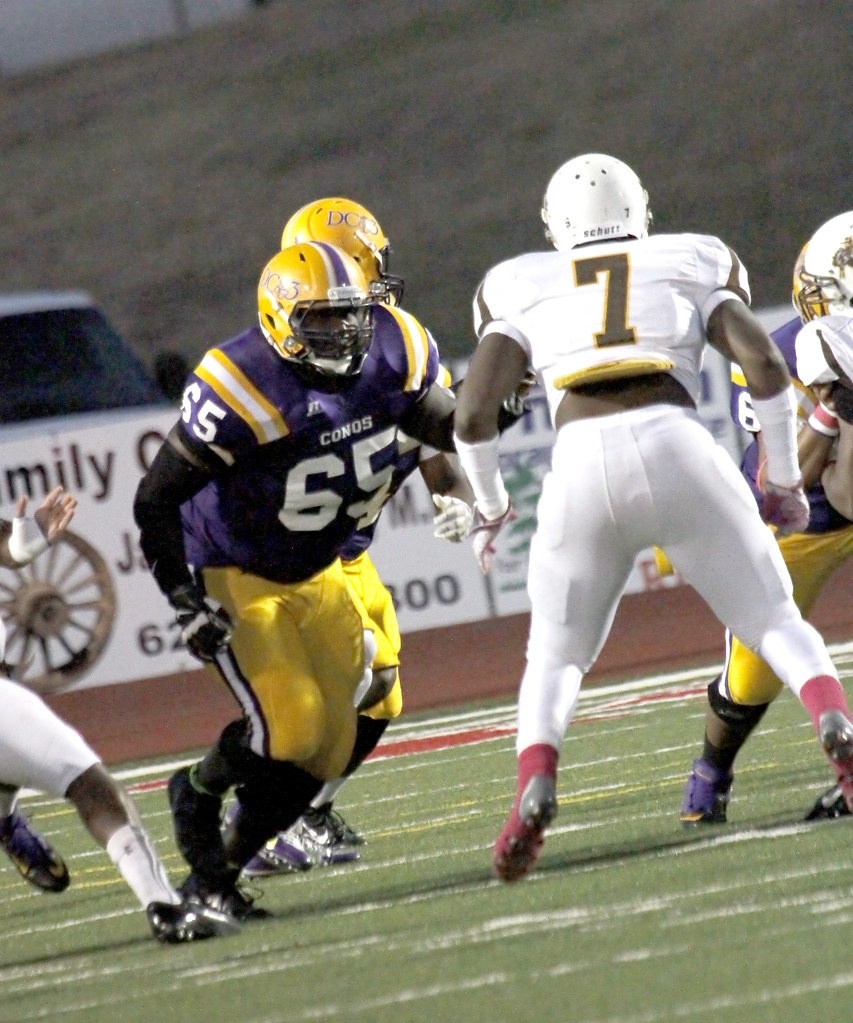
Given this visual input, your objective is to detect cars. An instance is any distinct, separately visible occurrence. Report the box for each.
[2,293,189,446]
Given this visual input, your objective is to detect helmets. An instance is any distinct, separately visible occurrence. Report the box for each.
[540,153,654,250]
[256,240,376,377]
[283,197,404,305]
[801,210,852,311]
[791,242,830,322]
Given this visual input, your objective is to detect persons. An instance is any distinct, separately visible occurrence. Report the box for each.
[222,196,472,880]
[451,153,853,887]
[0,486,241,943]
[132,240,537,922]
[679,211,853,824]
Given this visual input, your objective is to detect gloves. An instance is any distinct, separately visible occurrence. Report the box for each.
[167,586,233,663]
[431,492,472,543]
[500,369,538,415]
[759,481,811,541]
[467,500,520,574]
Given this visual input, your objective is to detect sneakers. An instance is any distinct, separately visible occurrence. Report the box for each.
[167,767,227,879]
[494,774,557,881]
[1,801,70,892]
[820,711,853,811]
[217,797,367,878]
[179,880,272,922]
[148,900,239,942]
[681,759,732,826]
